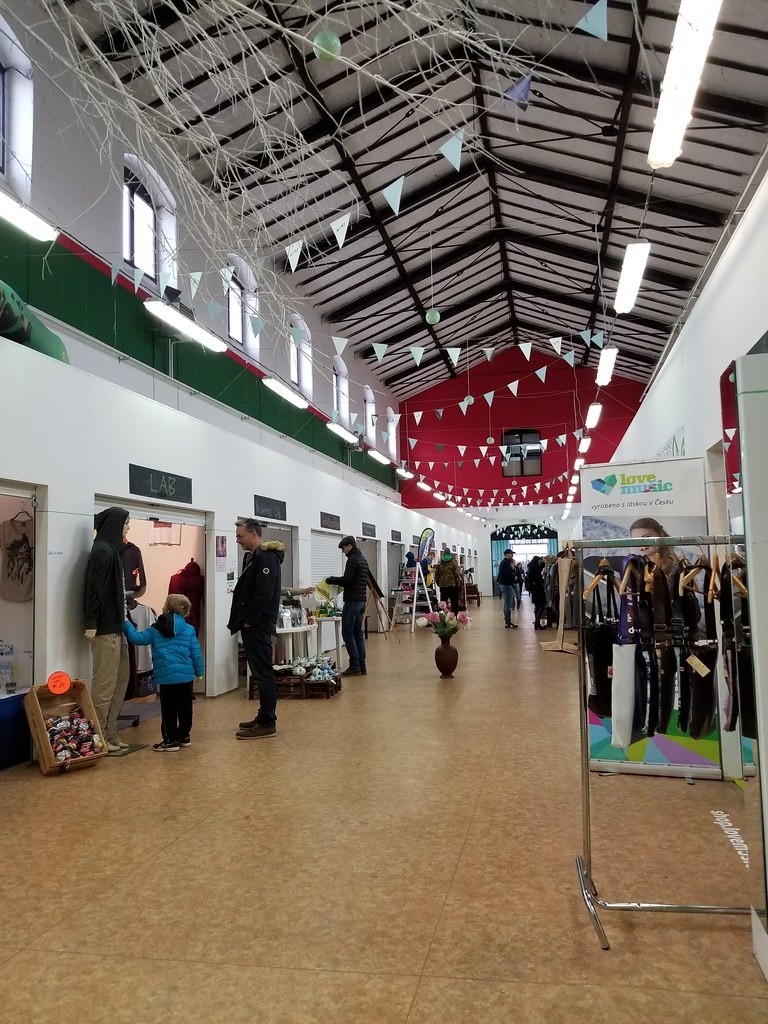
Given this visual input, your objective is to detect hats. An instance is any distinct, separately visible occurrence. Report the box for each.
[504,549,515,554]
[338,536,355,549]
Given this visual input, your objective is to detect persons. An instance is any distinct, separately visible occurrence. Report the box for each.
[84,506,130,751]
[497,548,525,628]
[125,594,203,751]
[122,536,146,599]
[326,536,368,676]
[421,552,436,590]
[281,586,315,596]
[527,556,546,631]
[436,548,462,621]
[583,518,708,719]
[227,519,285,739]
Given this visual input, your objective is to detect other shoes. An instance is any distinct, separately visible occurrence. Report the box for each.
[236,724,276,740]
[360,668,367,675]
[342,668,362,676]
[512,607,514,610]
[239,715,276,730]
[505,623,519,628]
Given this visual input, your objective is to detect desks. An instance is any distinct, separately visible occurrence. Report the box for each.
[272,624,318,664]
[316,618,342,668]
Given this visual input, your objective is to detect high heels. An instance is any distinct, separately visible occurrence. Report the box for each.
[535,624,546,630]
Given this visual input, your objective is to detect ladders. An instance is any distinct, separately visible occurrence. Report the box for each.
[390,561,434,633]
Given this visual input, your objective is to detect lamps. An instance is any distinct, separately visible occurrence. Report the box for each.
[417,481,486,522]
[395,467,414,478]
[613,238,651,314]
[143,297,228,352]
[367,448,391,465]
[578,436,591,452]
[262,376,309,409]
[647,0,723,169]
[595,344,619,387]
[326,420,360,443]
[585,402,602,429]
[0,184,60,242]
[561,457,584,520]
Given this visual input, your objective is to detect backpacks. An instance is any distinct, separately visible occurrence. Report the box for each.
[525,572,535,591]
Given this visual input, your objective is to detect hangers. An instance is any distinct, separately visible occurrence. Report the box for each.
[11,499,34,521]
[730,553,748,598]
[583,535,729,603]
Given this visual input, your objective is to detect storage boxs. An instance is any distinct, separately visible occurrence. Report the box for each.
[25,678,108,776]
[248,661,343,700]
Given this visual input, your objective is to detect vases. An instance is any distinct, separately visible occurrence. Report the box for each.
[434,636,458,679]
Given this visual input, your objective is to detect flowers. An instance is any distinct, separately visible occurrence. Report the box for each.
[415,601,473,636]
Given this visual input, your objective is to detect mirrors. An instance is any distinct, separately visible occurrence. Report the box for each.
[720,359,768,936]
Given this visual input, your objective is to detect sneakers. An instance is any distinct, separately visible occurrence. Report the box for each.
[177,734,192,746]
[152,738,180,752]
